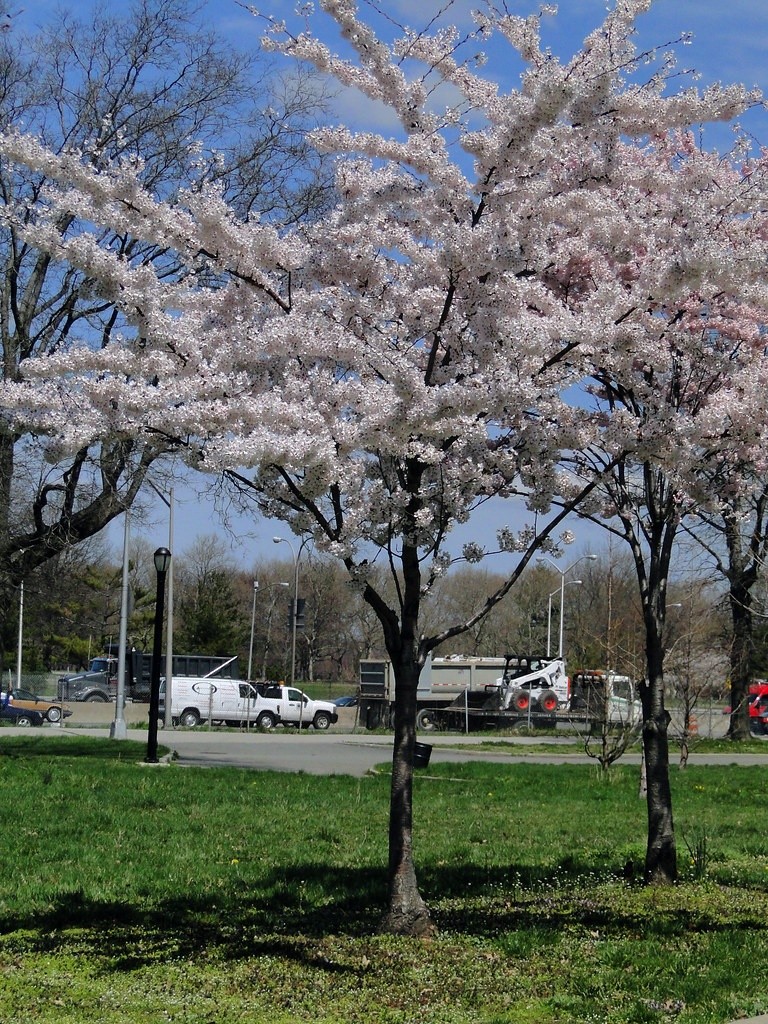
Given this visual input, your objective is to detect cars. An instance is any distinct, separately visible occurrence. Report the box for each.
[158,677,224,726]
[0,700,44,727]
[9,688,73,723]
[749,684,768,735]
[334,697,358,707]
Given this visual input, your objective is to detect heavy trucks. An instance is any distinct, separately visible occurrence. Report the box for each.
[358,647,644,738]
[57,645,237,702]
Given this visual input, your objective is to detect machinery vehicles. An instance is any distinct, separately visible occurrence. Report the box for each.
[446,654,572,712]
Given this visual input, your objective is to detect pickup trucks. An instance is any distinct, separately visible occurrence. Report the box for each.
[259,687,338,731]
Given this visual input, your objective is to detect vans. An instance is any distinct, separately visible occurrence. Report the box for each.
[171,677,280,731]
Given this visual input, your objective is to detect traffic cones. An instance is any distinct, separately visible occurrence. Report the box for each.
[689,716,698,736]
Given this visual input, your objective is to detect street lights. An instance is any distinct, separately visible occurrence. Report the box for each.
[273,537,315,684]
[246,582,288,681]
[537,555,597,658]
